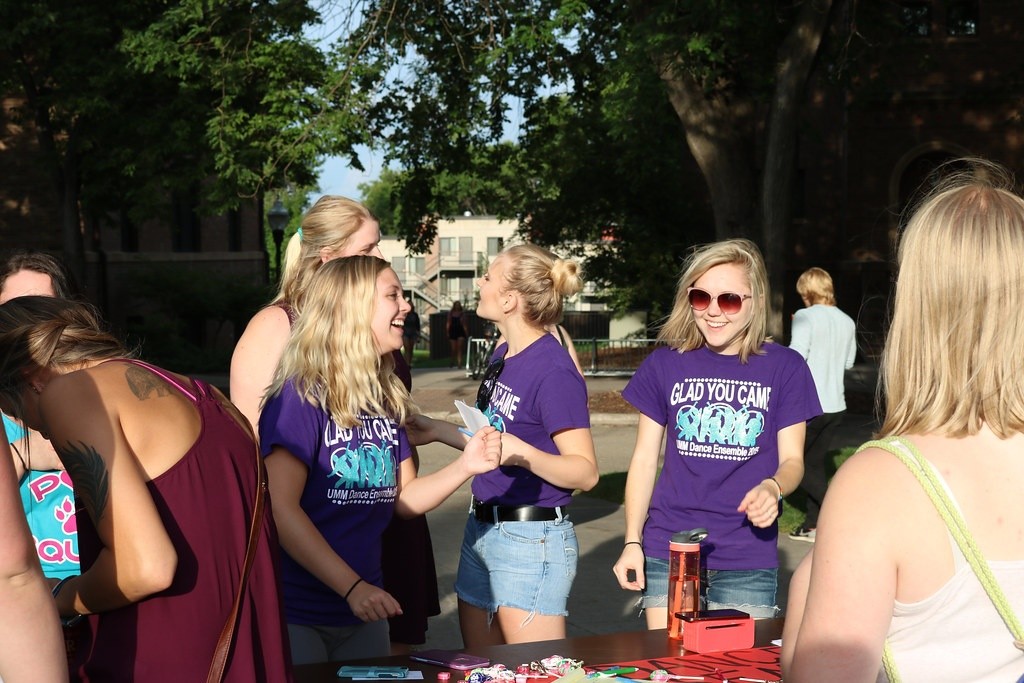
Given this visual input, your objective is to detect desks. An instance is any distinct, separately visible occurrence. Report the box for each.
[292,618,784,683]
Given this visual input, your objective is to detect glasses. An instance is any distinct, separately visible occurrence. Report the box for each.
[686,287,751,315]
[477,356,504,409]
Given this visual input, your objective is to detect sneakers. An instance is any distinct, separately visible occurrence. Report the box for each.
[788,527,816,542]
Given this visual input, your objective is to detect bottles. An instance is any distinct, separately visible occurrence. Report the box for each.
[667,528,709,641]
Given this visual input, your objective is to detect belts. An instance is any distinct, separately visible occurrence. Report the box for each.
[472,498,566,522]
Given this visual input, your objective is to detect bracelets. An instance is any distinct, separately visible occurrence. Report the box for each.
[762,477,783,501]
[623,541,641,549]
[50,575,83,628]
[344,576,368,600]
[10,442,27,470]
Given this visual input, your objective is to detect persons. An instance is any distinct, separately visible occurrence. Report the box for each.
[447,302,469,369]
[227,196,385,448]
[403,297,421,363]
[406,244,599,651]
[611,239,824,632]
[782,186,1024,683]
[0,253,289,683]
[258,257,503,662]
[785,266,857,543]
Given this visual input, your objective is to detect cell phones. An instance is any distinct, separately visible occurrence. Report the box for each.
[409,649,490,670]
[337,665,409,678]
[674,609,750,622]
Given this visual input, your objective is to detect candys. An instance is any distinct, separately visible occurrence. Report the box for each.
[464,654,706,683]
